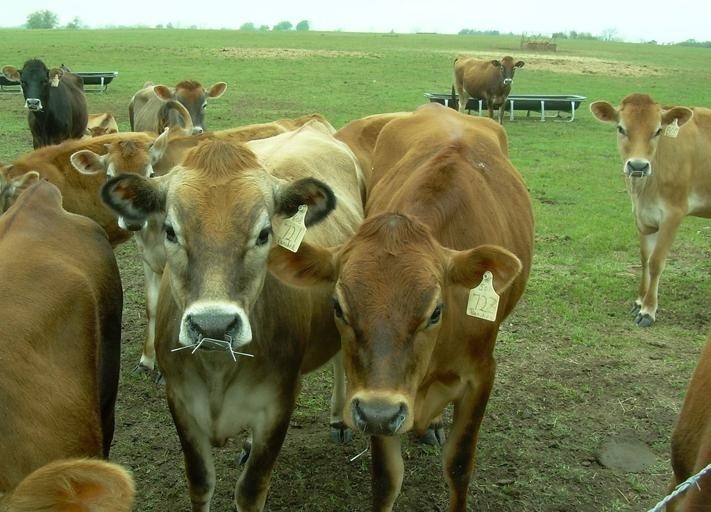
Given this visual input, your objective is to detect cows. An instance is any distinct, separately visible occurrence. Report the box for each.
[1,175,140,510]
[448,55,525,125]
[83,110,120,137]
[127,79,229,136]
[97,113,367,511]
[662,334,711,510]
[268,102,538,511]
[0,127,163,251]
[0,57,89,149]
[589,91,710,330]
[68,117,294,381]
